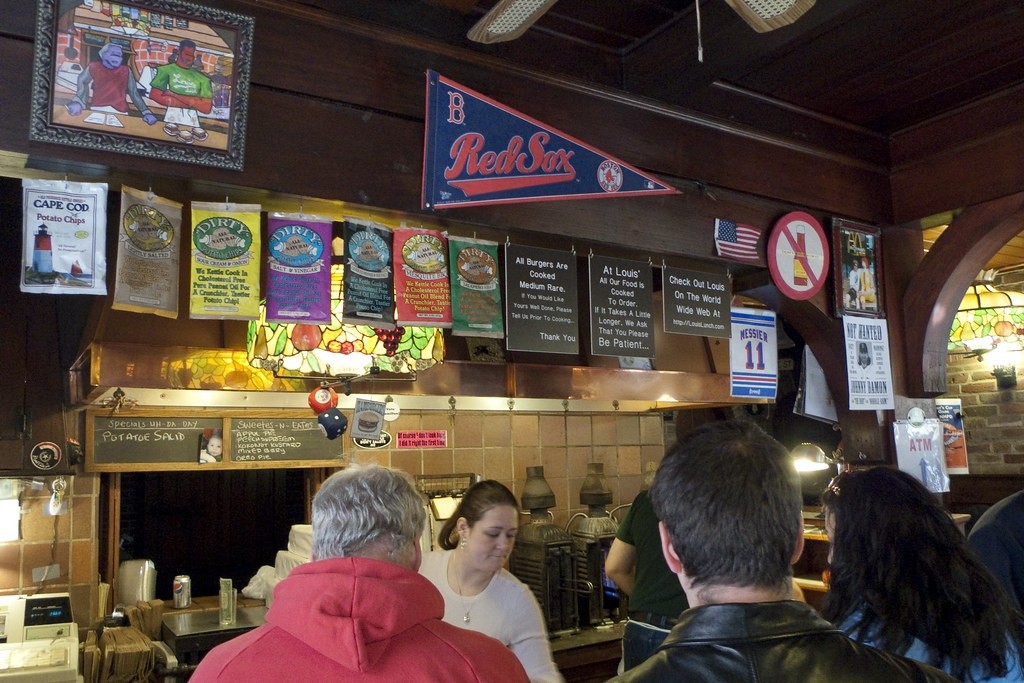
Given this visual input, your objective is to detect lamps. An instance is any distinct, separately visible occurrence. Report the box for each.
[169,348,310,392]
[246,224,446,397]
[792,444,831,472]
[990,364,1017,389]
[947,268,1024,362]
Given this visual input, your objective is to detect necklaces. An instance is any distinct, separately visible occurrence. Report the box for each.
[453,555,491,623]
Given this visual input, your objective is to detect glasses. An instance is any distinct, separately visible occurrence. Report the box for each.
[827,469,867,497]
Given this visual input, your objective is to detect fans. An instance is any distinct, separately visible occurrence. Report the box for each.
[466,0,817,45]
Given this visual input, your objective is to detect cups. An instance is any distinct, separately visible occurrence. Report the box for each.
[219,588,237,625]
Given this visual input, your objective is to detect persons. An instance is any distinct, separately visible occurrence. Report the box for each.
[858,343,872,370]
[604,491,690,675]
[821,466,1024,683]
[187,464,531,683]
[607,420,963,683]
[418,480,565,683]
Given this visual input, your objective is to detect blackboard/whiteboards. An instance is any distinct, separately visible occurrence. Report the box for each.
[661,265,732,339]
[82,404,353,470]
[504,240,581,355]
[586,252,659,360]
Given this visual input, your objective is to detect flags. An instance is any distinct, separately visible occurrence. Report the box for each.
[715,219,761,262]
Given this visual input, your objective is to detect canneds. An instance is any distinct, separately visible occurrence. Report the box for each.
[173,574,192,609]
